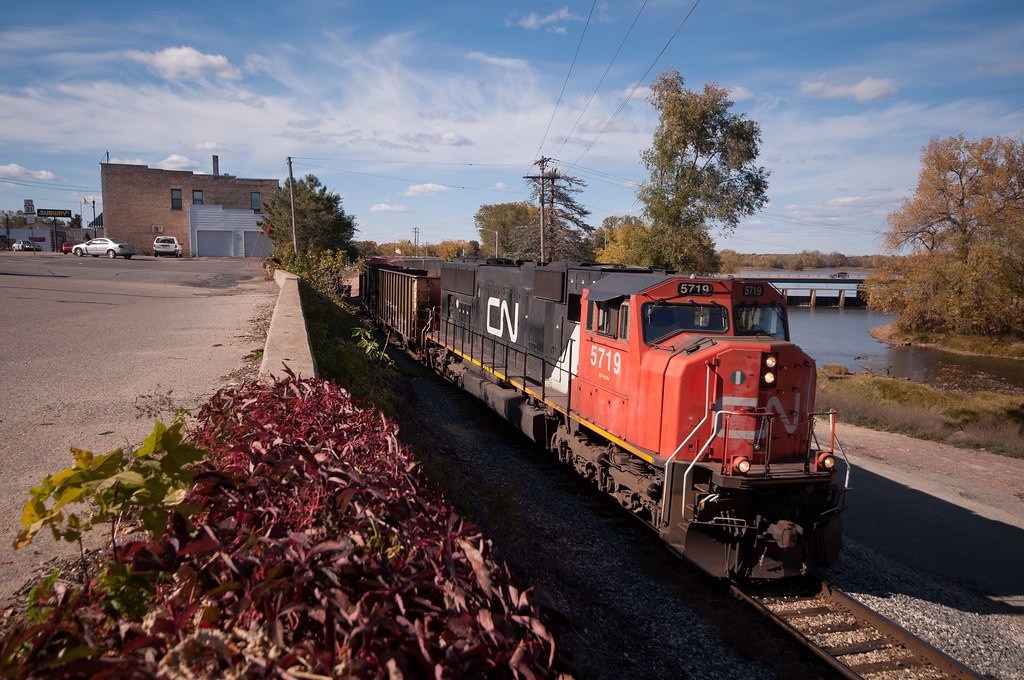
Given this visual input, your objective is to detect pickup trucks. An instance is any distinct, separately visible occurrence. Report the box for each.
[12,240,41,252]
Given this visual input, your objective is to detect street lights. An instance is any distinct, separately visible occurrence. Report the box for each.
[5,213,9,248]
[91,196,96,238]
[478,228,498,258]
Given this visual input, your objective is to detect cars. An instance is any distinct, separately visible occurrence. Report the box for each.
[830,272,849,278]
[62,239,86,254]
[153,236,183,258]
[71,237,137,259]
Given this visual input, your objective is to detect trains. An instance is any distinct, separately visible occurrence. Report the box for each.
[359,256,855,579]
